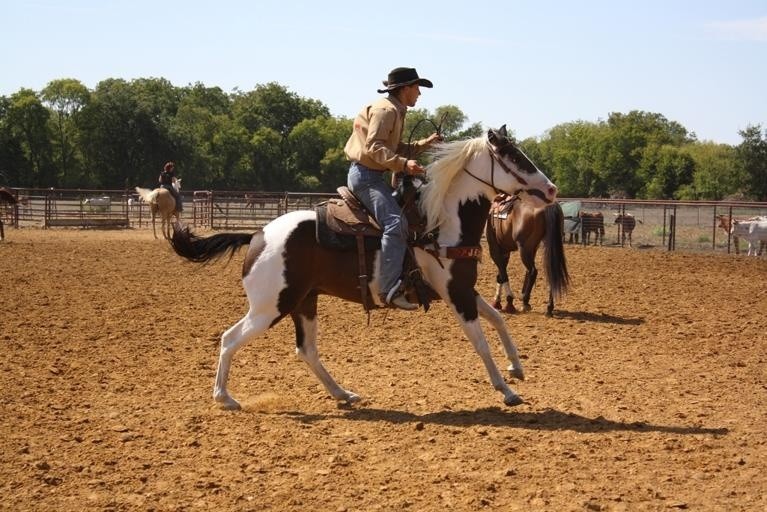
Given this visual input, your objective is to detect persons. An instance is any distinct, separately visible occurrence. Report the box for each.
[343,68,443,309]
[159,163,183,211]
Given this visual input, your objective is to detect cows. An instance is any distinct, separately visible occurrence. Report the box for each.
[615,214,642,248]
[244,192,266,213]
[128,198,135,211]
[715,215,767,256]
[83,196,111,218]
[579,210,605,247]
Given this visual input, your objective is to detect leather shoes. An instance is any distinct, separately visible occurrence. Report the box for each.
[380,295,418,310]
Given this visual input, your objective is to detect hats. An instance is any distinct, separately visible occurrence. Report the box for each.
[377,68,433,93]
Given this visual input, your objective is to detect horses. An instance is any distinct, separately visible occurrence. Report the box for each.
[0,186,29,241]
[486,190,572,317]
[135,177,182,239]
[163,125,556,410]
